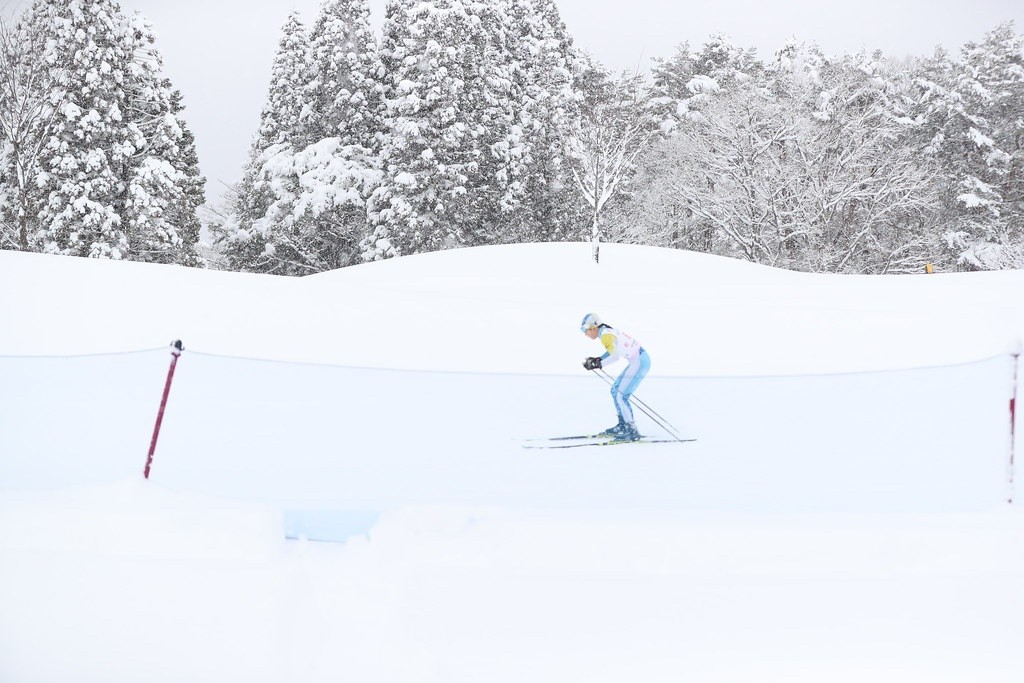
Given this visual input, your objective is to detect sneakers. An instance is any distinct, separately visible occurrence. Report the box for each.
[606,423,624,434]
[616,427,641,441]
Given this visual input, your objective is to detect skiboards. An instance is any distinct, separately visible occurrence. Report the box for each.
[510,433,699,450]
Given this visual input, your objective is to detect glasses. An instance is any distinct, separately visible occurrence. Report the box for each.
[581,326,587,333]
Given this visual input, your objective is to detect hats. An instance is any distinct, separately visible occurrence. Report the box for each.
[582,313,603,330]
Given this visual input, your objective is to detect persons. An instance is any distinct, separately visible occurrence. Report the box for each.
[579,311,651,439]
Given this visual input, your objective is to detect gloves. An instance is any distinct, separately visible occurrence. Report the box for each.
[583,357,602,371]
